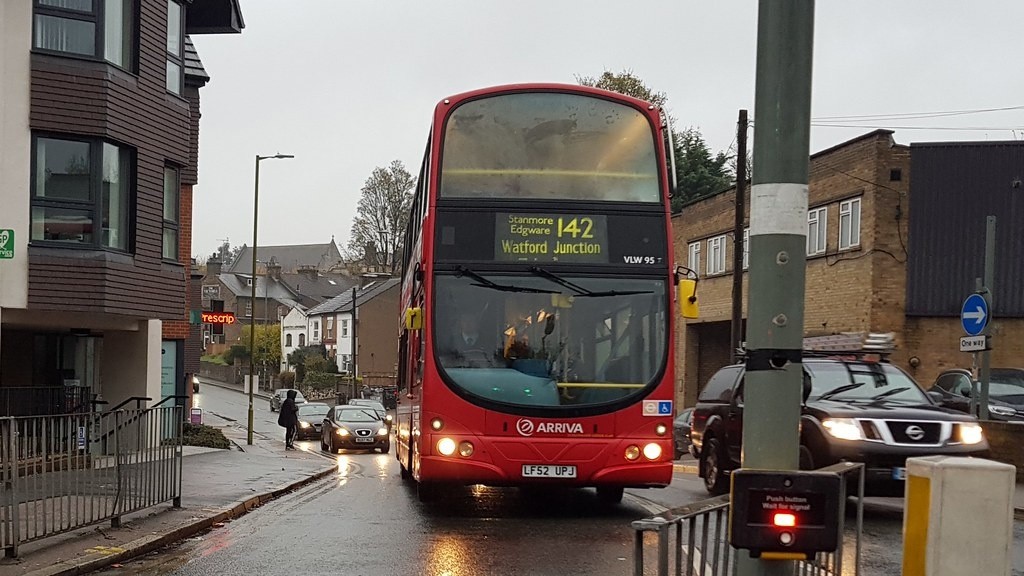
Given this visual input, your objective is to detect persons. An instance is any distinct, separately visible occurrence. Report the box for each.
[280,390,299,452]
[440,310,494,368]
[506,331,535,359]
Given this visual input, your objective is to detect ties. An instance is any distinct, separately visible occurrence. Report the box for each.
[467,338,473,347]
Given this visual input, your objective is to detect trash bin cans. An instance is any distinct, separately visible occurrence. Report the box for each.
[190,407,203,425]
[361,387,397,410]
[902,454,1018,576]
[335,392,346,406]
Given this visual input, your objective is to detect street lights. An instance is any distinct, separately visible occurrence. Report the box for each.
[247,154,295,446]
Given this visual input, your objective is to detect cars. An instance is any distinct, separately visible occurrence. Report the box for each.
[270,388,307,412]
[673,407,695,461]
[320,405,390,453]
[295,403,330,440]
[193,377,199,393]
[348,399,392,431]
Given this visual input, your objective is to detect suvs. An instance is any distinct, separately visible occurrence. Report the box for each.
[692,329,991,497]
[926,367,1024,422]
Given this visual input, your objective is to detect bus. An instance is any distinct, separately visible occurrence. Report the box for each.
[394,83,702,506]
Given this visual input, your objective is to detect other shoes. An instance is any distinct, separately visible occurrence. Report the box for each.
[286,447,296,451]
